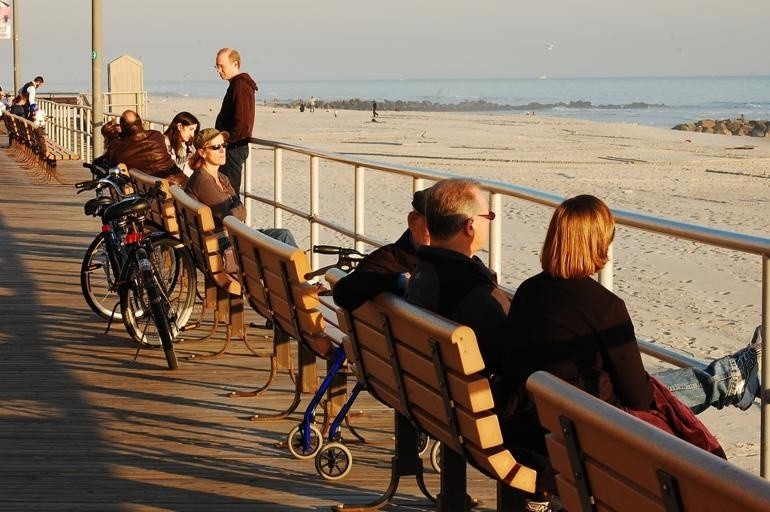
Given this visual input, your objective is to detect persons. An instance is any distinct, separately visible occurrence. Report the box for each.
[331,188,433,313]
[214,50,258,196]
[90,111,189,190]
[162,111,194,179]
[185,127,299,253]
[490,194,763,495]
[0,76,46,135]
[372,100,379,118]
[406,177,512,368]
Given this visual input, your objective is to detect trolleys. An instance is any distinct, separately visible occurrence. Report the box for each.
[286,241,441,481]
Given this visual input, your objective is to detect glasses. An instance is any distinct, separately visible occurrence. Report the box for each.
[202,142,228,150]
[478,211,496,220]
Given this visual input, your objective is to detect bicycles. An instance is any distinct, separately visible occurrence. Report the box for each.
[74,162,180,324]
[74,178,198,372]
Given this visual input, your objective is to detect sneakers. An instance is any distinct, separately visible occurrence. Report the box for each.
[733,324,764,411]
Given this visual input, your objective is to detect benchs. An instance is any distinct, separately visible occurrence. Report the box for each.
[525,371,770,512]
[26,123,80,185]
[325,267,536,511]
[168,185,275,360]
[110,164,133,198]
[3,112,11,151]
[223,216,396,448]
[130,169,180,240]
[13,117,52,169]
[7,115,18,159]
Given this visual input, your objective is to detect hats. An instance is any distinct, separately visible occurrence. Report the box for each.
[411,186,433,215]
[101,119,122,136]
[193,128,231,149]
[29,103,39,112]
